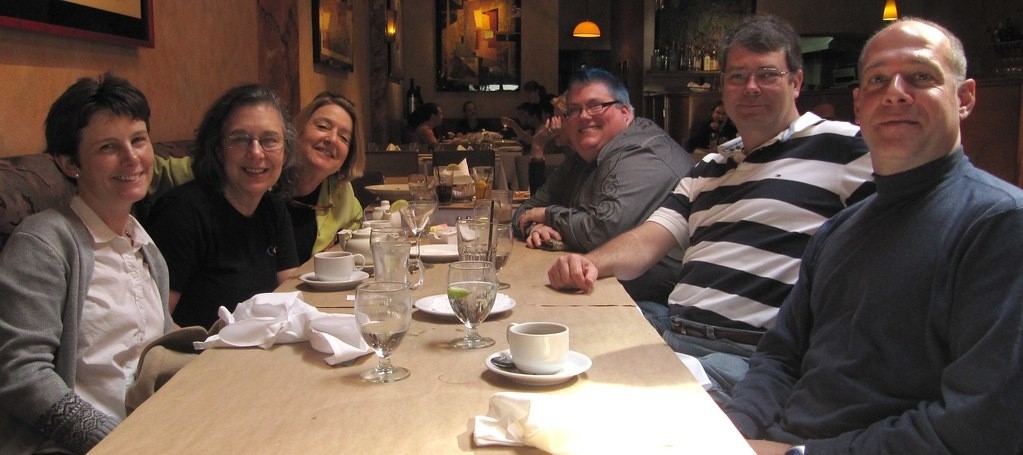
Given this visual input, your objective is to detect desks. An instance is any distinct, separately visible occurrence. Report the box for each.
[74,191,758,455]
[420,151,523,191]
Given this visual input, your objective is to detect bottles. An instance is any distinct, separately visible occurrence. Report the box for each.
[651,40,721,72]
[985,17,1023,47]
[407,78,423,119]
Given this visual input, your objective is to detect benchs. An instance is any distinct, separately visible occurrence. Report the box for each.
[0,138,197,249]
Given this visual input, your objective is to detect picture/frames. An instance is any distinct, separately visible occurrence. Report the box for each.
[387,0,405,82]
[309,0,354,73]
[434,0,521,93]
[0,0,154,50]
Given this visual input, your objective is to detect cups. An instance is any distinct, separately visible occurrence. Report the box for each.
[506,322,569,375]
[363,220,392,229]
[433,166,453,205]
[370,227,425,290]
[408,173,439,237]
[457,199,501,282]
[501,114,509,131]
[719,136,728,144]
[473,166,494,199]
[313,253,366,281]
[491,190,512,224]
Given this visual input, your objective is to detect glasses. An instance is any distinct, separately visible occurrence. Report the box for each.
[720,65,791,89]
[563,100,622,120]
[220,133,287,151]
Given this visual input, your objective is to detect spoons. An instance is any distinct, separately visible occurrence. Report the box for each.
[491,357,515,368]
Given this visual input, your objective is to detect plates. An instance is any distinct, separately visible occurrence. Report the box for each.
[415,293,516,321]
[485,349,592,385]
[299,265,375,290]
[408,244,460,262]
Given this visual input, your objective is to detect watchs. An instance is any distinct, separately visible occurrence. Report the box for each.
[784,446,805,455]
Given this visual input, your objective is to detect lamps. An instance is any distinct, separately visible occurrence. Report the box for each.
[573,0,601,37]
[883,0,903,21]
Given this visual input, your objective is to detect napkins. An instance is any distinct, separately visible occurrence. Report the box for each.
[427,157,474,189]
[191,290,377,368]
[473,383,556,455]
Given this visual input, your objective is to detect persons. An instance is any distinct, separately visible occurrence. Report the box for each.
[548,12,873,408]
[512,67,698,335]
[101,90,369,265]
[405,80,573,197]
[0,85,183,455]
[130,83,301,331]
[796,94,841,120]
[723,17,1023,455]
[686,102,739,153]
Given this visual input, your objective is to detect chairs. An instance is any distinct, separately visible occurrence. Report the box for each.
[364,152,419,177]
[514,156,530,191]
[431,149,496,188]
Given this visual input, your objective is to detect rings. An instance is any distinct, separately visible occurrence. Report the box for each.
[531,230,541,237]
[525,210,527,215]
[548,128,553,135]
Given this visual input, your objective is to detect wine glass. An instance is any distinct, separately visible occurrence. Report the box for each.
[354,282,412,384]
[398,202,438,271]
[476,227,513,290]
[447,261,497,349]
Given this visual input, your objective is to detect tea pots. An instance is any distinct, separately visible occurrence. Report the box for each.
[337,227,395,265]
[372,200,402,229]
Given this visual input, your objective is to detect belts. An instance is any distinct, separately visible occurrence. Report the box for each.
[670,319,764,347]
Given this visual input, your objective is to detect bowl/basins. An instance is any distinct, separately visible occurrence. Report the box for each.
[451,184,475,202]
